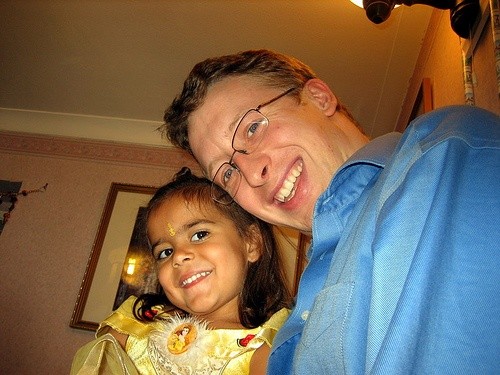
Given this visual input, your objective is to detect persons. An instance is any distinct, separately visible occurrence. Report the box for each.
[70,166,296,375]
[155,48,500,375]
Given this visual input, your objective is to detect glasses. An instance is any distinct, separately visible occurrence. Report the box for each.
[209,85,299,205]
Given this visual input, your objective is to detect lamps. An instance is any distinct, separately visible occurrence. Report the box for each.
[350,0,481,42]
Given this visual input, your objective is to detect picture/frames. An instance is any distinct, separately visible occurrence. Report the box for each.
[68,182,310,332]
[405,78,433,131]
